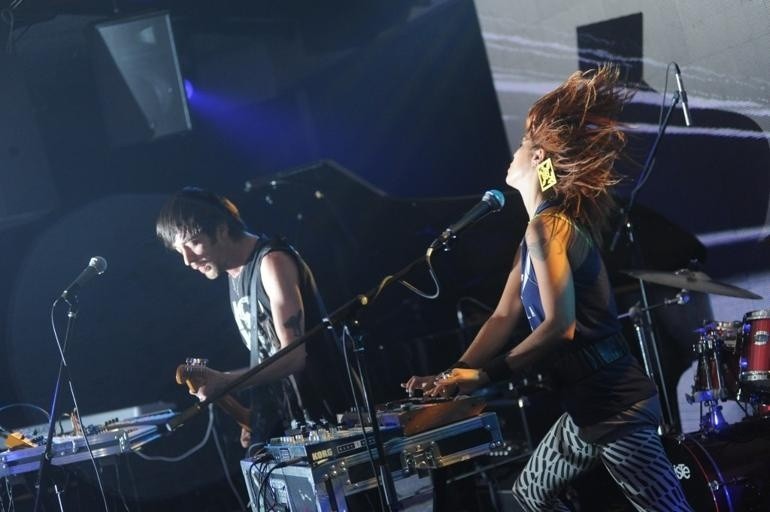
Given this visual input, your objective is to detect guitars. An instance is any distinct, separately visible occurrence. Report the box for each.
[176,358,274,451]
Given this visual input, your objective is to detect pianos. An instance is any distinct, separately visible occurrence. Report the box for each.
[0,401,182,448]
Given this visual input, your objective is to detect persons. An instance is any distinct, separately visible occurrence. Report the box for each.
[400,62,695,512]
[155,186,362,449]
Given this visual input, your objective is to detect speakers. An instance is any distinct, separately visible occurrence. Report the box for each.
[91,9,192,150]
[0,51,59,230]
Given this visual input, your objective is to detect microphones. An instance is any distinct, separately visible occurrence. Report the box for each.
[673,66,695,127]
[429,188,506,257]
[53,257,108,305]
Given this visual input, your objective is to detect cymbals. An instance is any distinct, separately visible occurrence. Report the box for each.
[620,263,763,300]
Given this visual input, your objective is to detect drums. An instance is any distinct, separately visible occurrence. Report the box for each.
[665,430,735,511]
[693,320,742,400]
[737,309,769,397]
[756,393,770,422]
